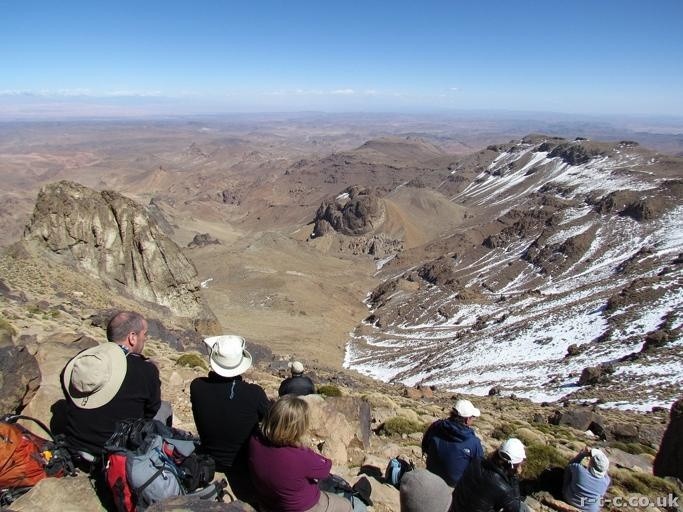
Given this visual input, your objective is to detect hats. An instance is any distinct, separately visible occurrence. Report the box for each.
[591,449,609,472]
[287,361,305,373]
[204,335,253,379]
[62,342,128,409]
[453,399,481,418]
[498,438,527,464]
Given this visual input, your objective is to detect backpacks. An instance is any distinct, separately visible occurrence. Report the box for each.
[97,419,226,511]
[0,413,76,506]
[385,457,415,490]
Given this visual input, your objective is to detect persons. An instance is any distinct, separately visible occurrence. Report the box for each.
[249,394,353,512]
[421,399,484,488]
[447,438,531,512]
[278,361,314,397]
[539,446,611,512]
[190,335,269,498]
[64,312,172,474]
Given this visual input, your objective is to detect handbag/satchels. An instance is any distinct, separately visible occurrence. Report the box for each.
[317,471,352,493]
